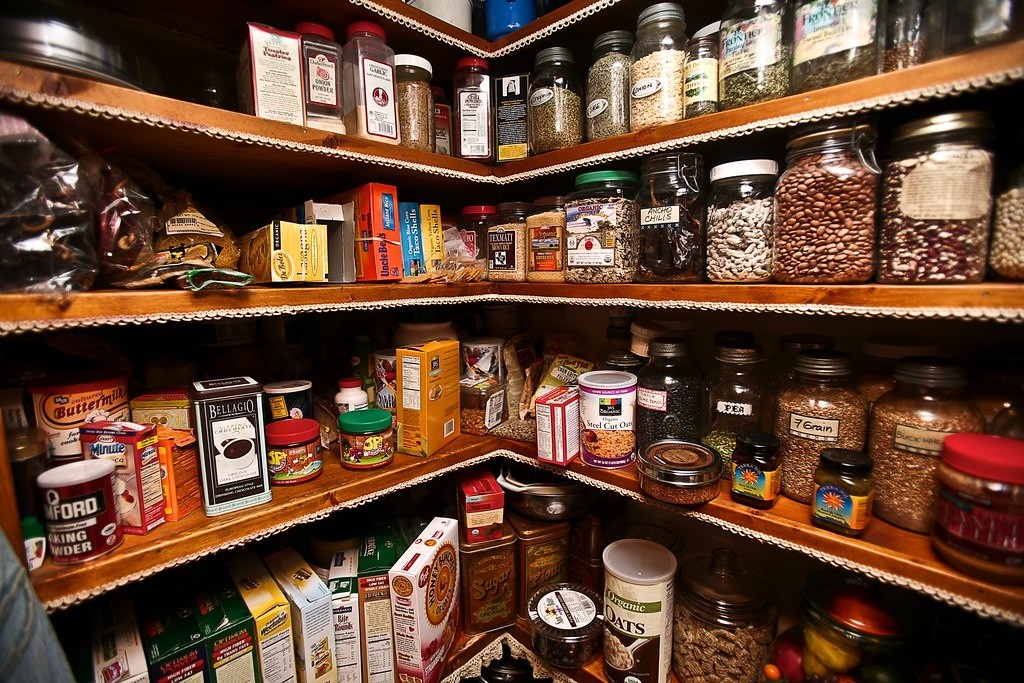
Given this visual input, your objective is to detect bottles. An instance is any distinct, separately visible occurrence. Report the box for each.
[335,378,368,419]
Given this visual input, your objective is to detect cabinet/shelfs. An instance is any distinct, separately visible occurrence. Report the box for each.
[1,0,1024,683]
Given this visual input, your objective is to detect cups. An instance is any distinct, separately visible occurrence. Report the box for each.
[222,438,256,469]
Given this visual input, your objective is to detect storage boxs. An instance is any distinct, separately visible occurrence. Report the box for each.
[130,388,193,427]
[458,467,505,543]
[495,73,529,163]
[141,593,210,682]
[318,181,402,281]
[328,546,363,683]
[396,338,460,458]
[238,220,329,284]
[266,200,357,284]
[235,22,307,128]
[157,426,202,523]
[90,599,152,683]
[388,517,463,683]
[223,547,299,683]
[262,548,338,683]
[79,421,166,536]
[190,570,261,683]
[357,524,396,683]
[536,386,581,466]
[420,205,445,277]
[191,375,273,518]
[399,202,426,279]
